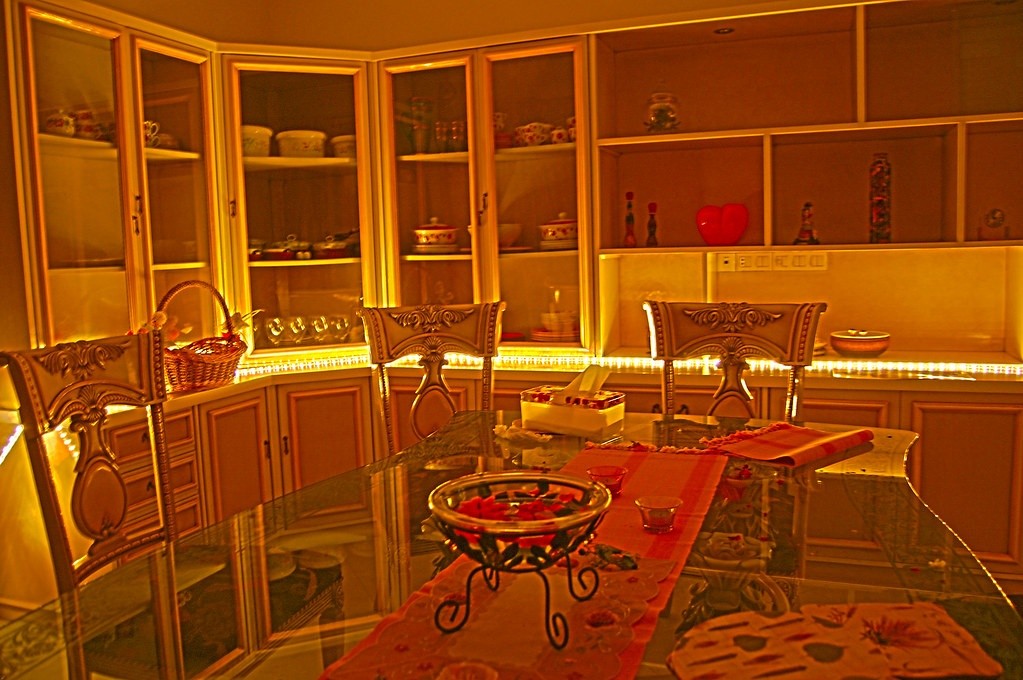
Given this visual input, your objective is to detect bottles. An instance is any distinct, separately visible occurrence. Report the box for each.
[867,152,892,243]
[646,203,658,247]
[623,191,636,247]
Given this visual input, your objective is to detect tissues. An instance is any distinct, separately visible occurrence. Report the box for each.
[517,364,625,431]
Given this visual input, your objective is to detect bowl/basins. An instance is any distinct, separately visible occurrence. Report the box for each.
[634,496,683,533]
[275,130,326,158]
[330,134,356,156]
[540,310,577,331]
[536,212,577,240]
[585,464,628,497]
[465,223,521,245]
[241,125,274,157]
[830,328,890,357]
[427,469,611,567]
[409,218,458,244]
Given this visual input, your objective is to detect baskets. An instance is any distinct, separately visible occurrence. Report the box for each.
[156,280,249,394]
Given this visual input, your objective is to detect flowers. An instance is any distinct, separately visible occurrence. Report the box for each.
[221,334,241,354]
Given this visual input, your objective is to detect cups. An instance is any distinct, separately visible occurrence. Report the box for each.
[40,107,161,148]
[400,96,464,152]
[550,117,576,143]
[493,112,506,131]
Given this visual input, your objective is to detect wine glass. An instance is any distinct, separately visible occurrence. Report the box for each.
[252,314,351,348]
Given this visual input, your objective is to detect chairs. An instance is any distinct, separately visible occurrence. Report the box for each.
[355,301,505,459]
[642,301,829,422]
[0,329,347,680]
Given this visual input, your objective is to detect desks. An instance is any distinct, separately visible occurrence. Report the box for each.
[0,408,1023,680]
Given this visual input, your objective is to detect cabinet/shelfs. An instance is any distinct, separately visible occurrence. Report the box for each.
[0,1,1023,622]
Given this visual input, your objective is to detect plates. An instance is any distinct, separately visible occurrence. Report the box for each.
[60,254,124,266]
[502,326,579,344]
[812,339,828,355]
[512,122,553,145]
[410,240,577,253]
